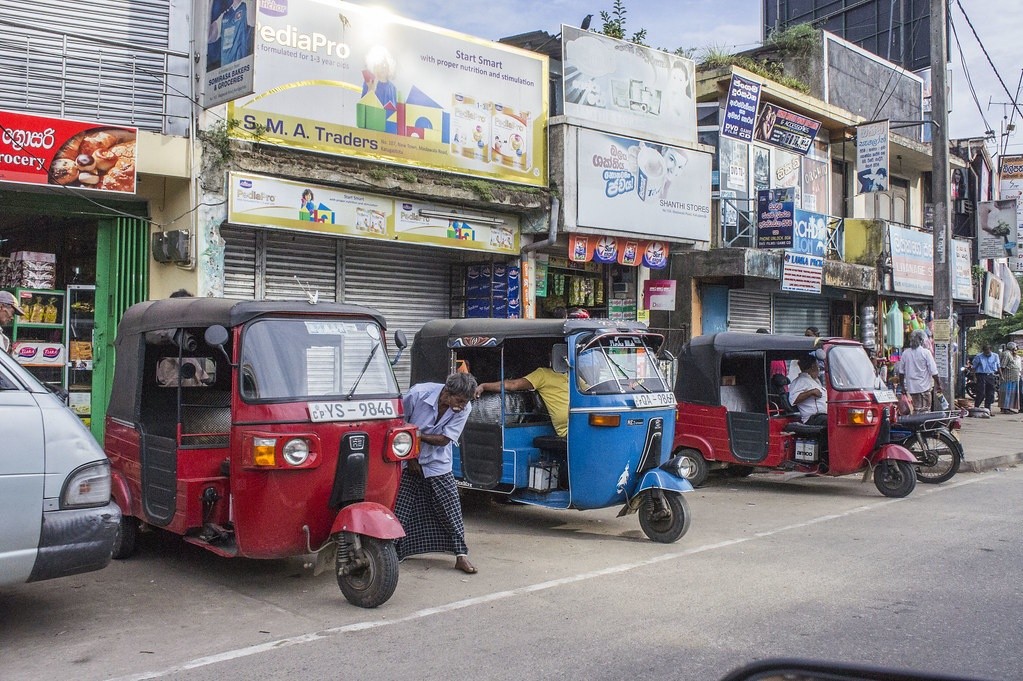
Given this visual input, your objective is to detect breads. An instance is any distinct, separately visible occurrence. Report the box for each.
[46,126,136,191]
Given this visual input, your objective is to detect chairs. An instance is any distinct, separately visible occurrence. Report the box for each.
[773,374,829,439]
[531,392,570,452]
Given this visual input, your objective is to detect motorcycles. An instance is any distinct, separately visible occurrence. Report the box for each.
[408,317,696,544]
[671,331,924,500]
[102,297,422,608]
[895,409,966,485]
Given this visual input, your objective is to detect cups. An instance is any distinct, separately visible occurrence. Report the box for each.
[637,140,667,202]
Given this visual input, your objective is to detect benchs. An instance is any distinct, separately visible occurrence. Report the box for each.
[463,388,554,426]
[721,383,769,414]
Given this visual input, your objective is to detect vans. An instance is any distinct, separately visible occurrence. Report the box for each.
[0,347,123,586]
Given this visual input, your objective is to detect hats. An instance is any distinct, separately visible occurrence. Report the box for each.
[0,290,25,317]
[982,340,992,350]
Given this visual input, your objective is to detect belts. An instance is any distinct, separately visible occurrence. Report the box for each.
[976,373,993,376]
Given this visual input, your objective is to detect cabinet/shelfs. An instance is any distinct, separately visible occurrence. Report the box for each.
[0,286,67,400]
[66,283,96,419]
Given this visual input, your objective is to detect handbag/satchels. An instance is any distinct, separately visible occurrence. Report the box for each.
[898,394,910,416]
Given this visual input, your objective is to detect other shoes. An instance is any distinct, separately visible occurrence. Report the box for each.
[820,454,829,472]
[558,462,568,491]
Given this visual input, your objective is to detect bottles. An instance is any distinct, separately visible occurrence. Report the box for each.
[903,303,916,321]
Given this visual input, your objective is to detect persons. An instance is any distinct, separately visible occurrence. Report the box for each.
[754,327,850,466]
[0,291,26,354]
[156,288,216,387]
[899,329,1023,417]
[300,189,315,220]
[566,310,603,386]
[858,168,887,192]
[951,168,966,197]
[389,374,480,574]
[663,62,692,122]
[755,105,777,143]
[475,343,591,490]
[628,142,689,210]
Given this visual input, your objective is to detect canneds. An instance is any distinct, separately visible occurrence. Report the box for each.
[638,148,666,203]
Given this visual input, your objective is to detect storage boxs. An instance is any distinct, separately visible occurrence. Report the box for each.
[0,251,56,289]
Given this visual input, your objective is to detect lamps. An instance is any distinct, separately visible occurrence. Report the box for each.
[418,208,504,226]
